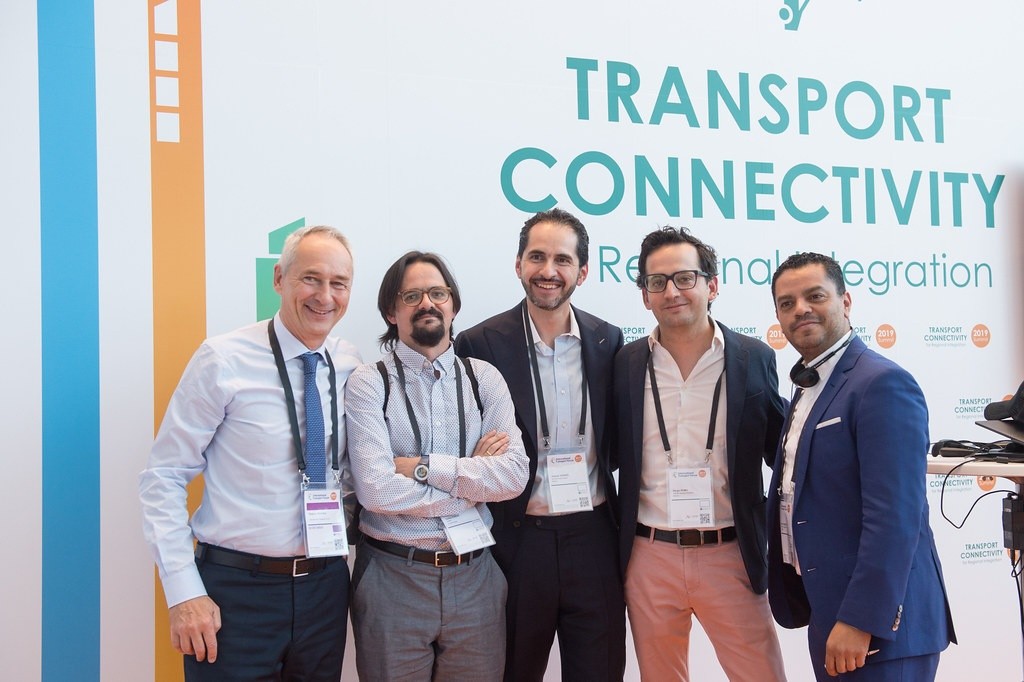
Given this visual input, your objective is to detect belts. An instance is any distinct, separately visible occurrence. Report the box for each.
[195,542,342,578]
[363,535,484,567]
[635,522,738,547]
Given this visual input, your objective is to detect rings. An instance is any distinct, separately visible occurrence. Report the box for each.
[486,451,492,456]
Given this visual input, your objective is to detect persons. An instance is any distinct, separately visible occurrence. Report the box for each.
[343,249,531,682]
[452,208,625,682]
[614,226,790,682]
[765,251,958,681]
[136,224,366,681]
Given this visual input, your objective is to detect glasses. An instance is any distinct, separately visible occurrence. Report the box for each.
[396,285,452,307]
[641,270,709,293]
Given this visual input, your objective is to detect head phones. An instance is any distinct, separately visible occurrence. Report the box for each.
[788,325,856,388]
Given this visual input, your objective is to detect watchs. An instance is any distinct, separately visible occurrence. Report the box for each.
[413,455,429,484]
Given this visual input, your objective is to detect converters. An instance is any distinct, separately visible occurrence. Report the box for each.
[1002,498,1024,549]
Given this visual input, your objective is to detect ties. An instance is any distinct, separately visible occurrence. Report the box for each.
[298,352,326,488]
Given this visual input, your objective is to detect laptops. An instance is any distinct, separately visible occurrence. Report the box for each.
[970,419,1024,463]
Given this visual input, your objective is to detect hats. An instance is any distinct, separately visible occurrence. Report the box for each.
[983,381,1024,426]
